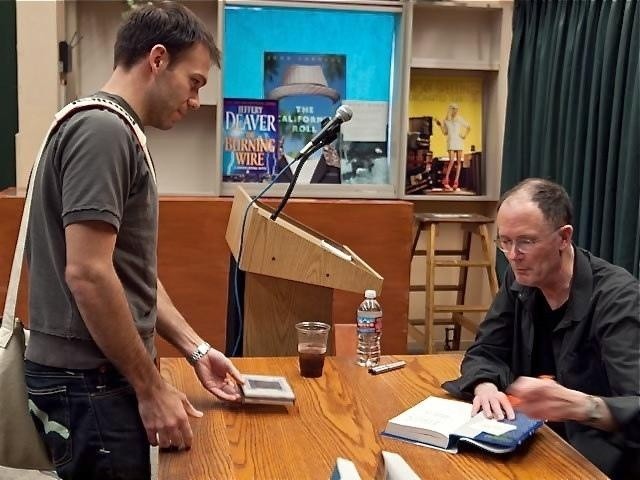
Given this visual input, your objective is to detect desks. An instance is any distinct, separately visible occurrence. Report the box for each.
[159,352,611,480]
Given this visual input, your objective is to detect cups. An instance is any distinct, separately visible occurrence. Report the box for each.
[297,321,330,378]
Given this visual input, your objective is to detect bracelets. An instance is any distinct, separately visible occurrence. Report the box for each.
[579,393,606,429]
[183,341,212,365]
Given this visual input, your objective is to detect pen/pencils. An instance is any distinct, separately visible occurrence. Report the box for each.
[368,360,406,375]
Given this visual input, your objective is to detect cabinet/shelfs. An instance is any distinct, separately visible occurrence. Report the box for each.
[57,0,513,198]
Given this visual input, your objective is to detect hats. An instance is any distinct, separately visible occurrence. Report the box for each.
[269,64,340,106]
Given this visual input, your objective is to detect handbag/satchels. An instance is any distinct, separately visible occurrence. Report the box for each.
[0,317,56,472]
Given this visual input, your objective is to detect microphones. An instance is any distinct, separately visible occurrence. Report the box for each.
[270,132,337,222]
[295,105,353,161]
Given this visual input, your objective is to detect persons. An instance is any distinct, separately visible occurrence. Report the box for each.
[433,104,471,191]
[22,3,244,479]
[441,176,640,479]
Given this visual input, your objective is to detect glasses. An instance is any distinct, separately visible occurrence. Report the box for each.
[493,226,564,255]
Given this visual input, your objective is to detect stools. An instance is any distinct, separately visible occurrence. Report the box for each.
[410,211,502,350]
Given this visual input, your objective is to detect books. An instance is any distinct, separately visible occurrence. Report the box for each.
[381,394,545,456]
[240,374,297,405]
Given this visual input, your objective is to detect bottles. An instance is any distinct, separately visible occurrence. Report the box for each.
[355,289,383,368]
